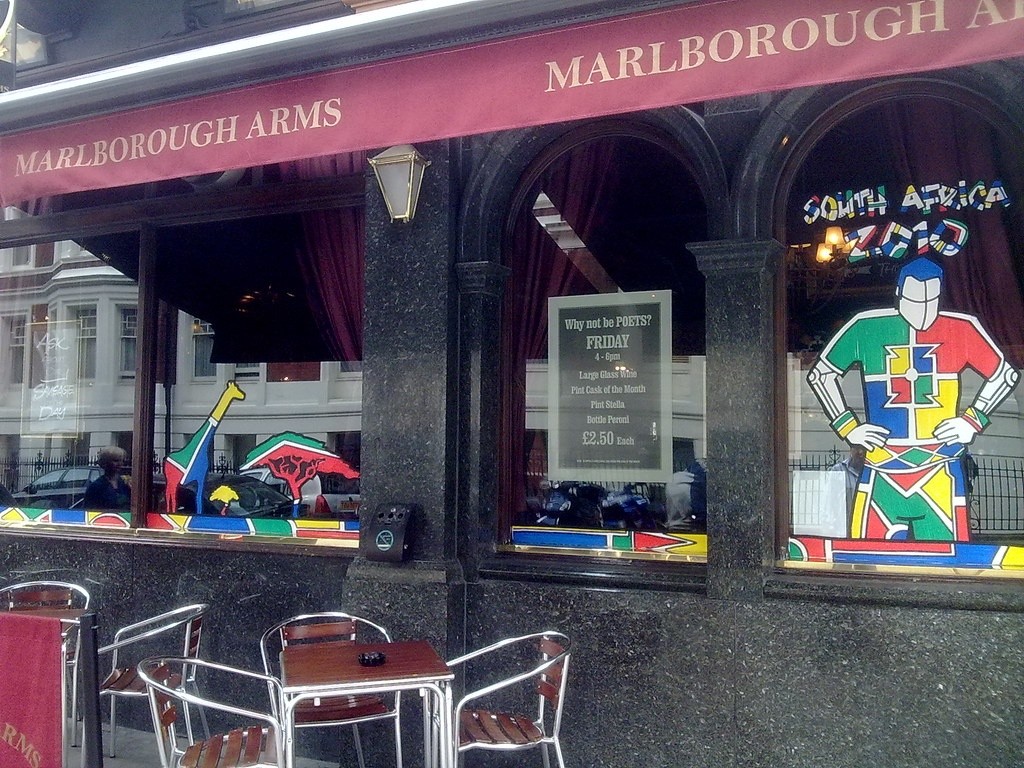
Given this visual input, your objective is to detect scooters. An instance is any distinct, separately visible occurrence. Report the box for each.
[601,481,664,533]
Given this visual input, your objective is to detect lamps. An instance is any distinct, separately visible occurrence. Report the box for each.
[789,233,812,267]
[367,142,434,226]
[817,226,846,270]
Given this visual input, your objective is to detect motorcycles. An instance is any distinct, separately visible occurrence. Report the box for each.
[534,477,604,533]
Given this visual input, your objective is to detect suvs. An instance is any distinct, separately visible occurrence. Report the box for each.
[239,468,361,521]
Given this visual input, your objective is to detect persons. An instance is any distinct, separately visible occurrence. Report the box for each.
[83,446,131,512]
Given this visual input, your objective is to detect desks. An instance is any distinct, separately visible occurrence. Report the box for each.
[278,639,456,768]
[12,607,90,746]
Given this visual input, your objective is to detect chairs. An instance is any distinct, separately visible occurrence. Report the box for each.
[0,581,573,768]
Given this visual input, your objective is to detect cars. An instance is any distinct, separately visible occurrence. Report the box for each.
[9,467,133,513]
[150,475,309,521]
